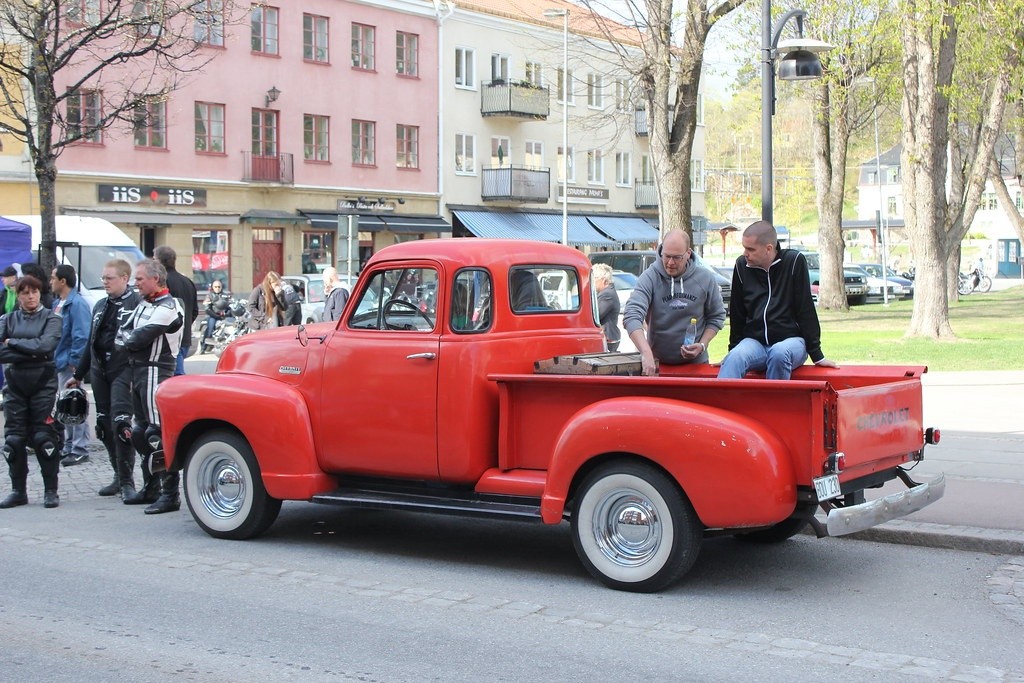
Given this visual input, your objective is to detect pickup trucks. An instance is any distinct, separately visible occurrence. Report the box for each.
[156,236,947,593]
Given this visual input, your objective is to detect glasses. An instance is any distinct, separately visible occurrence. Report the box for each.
[101,275,124,282]
[661,250,687,262]
[214,286,221,288]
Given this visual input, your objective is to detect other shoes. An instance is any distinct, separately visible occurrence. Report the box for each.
[59,449,89,465]
[200,349,205,354]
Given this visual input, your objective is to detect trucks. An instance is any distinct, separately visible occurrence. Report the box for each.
[0,214,147,328]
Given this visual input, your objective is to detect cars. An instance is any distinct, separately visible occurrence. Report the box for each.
[277,274,382,326]
[713,244,916,303]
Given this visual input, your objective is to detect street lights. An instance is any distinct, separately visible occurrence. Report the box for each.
[856,77,888,306]
[544,7,571,246]
[759,9,836,226]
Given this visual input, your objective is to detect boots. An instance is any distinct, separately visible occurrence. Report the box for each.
[99,457,119,496]
[116,456,137,504]
[44,476,59,508]
[123,462,160,504]
[0,478,28,507]
[144,471,180,514]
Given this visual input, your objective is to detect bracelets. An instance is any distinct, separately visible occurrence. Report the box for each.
[700,343,705,354]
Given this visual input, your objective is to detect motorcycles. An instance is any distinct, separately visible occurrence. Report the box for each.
[958,257,992,295]
[198,296,250,359]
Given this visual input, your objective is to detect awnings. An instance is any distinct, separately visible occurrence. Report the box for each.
[453,210,706,246]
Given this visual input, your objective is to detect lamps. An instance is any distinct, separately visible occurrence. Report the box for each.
[356,195,366,204]
[377,196,387,205]
[397,197,406,205]
[265,87,281,107]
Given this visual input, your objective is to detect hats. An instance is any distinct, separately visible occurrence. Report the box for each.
[0,266,17,276]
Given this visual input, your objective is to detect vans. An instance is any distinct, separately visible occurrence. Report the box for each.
[536,270,639,315]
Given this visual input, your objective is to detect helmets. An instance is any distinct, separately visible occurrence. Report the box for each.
[59,388,88,426]
[230,300,245,316]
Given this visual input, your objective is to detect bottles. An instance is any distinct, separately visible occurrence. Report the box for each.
[684,318,697,346]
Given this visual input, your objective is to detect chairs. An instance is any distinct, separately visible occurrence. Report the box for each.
[450,271,547,333]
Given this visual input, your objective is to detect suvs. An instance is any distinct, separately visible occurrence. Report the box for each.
[588,250,732,315]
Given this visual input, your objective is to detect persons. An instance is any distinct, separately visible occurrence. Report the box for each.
[623,229,726,376]
[591,263,621,353]
[247,271,301,335]
[199,280,236,354]
[321,267,351,323]
[475,268,547,332]
[0,244,202,514]
[716,221,840,381]
